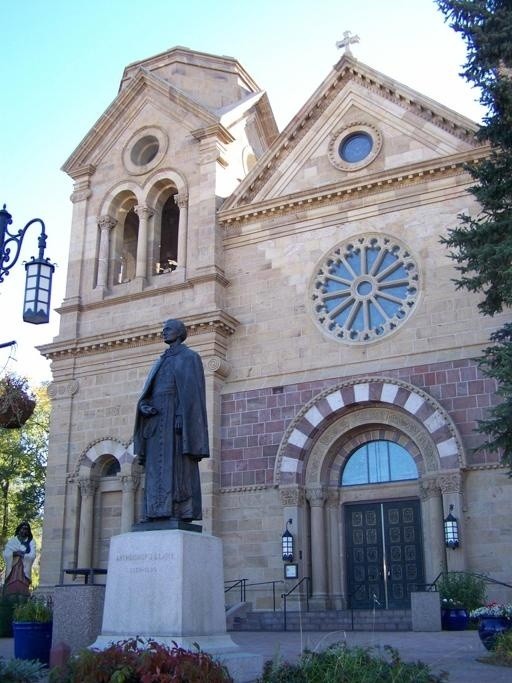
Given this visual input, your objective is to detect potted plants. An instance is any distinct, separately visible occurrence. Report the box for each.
[13,603,53,666]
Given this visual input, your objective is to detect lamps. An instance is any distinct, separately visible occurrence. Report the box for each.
[443,505,460,547]
[282,517,297,563]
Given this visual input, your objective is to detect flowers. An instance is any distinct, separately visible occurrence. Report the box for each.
[470,601,512,618]
[441,595,466,608]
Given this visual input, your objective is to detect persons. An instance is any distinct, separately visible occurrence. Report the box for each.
[133,319,209,522]
[3,522,36,596]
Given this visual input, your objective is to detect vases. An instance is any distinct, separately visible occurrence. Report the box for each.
[441,608,470,630]
[477,620,510,652]
